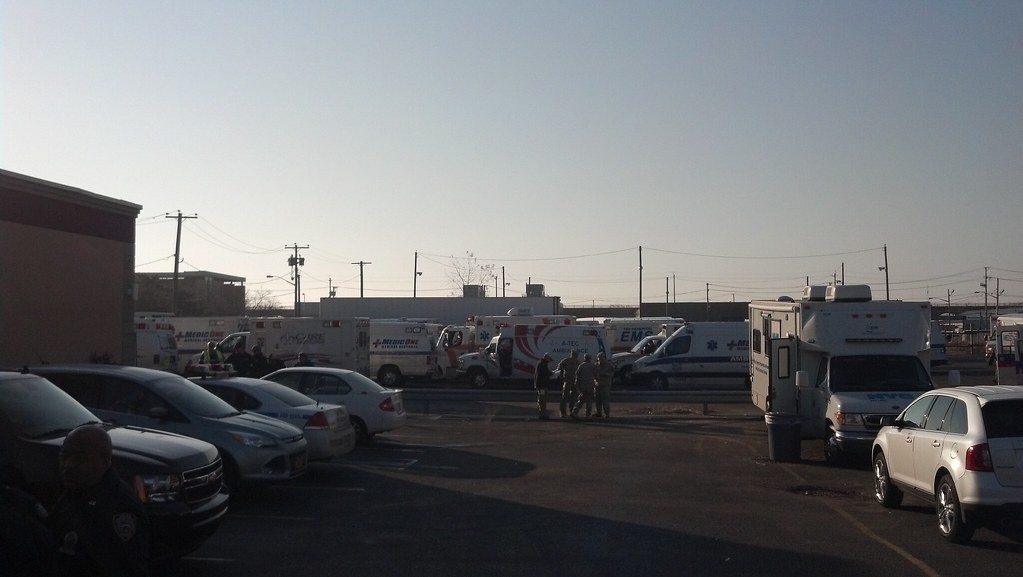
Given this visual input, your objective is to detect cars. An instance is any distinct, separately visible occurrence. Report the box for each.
[258,367,407,443]
[871,385,1023,543]
[184,376,358,471]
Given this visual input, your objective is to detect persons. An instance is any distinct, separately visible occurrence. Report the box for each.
[534,352,554,419]
[294,352,313,386]
[649,341,658,352]
[557,350,613,418]
[0,410,152,577]
[453,332,462,346]
[199,338,286,378]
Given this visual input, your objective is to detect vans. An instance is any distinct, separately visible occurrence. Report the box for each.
[925,319,947,353]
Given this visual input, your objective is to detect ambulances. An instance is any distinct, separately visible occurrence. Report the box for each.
[630,321,751,392]
[456,323,611,392]
[576,316,685,354]
[136,330,181,375]
[135,310,247,370]
[611,323,686,387]
[191,315,356,377]
[435,307,577,386]
[356,317,462,389]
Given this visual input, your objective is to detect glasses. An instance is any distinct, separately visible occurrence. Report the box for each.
[56,451,105,467]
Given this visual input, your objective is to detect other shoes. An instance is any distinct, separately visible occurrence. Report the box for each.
[538,414,610,421]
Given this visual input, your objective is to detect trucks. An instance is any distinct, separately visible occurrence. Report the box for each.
[747,284,940,469]
[984,324,1023,366]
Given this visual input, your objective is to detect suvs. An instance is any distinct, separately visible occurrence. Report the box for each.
[7,364,309,503]
[0,371,231,577]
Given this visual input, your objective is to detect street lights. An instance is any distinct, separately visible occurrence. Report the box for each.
[266,274,298,317]
[929,288,955,331]
[974,278,1005,386]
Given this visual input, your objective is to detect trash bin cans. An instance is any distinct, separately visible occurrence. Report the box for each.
[765,412,801,462]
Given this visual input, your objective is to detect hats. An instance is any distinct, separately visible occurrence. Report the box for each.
[596,352,606,361]
[545,352,553,361]
[571,349,581,354]
[584,353,591,360]
